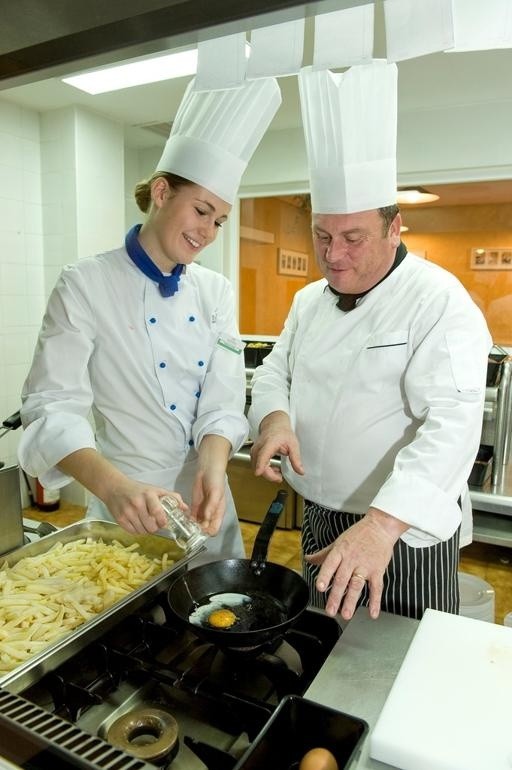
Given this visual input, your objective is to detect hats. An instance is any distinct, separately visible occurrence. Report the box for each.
[155,75,282,205]
[297,63,398,215]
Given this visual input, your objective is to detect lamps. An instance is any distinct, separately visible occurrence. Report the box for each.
[394,185,442,204]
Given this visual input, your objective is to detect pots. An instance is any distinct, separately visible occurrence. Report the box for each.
[168,492,312,643]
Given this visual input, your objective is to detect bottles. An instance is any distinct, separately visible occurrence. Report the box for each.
[155,497,206,555]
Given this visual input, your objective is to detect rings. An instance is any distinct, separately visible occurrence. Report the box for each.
[354,574,368,582]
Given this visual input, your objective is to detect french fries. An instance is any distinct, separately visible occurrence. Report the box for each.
[0,539,176,680]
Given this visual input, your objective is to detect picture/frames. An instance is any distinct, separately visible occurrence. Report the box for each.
[276,243,310,278]
[470,246,512,269]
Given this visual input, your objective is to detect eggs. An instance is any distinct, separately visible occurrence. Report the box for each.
[300,747,339,769]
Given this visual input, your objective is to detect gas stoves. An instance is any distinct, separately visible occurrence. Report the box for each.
[5,595,345,770]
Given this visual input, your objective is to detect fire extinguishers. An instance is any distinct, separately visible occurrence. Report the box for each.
[22,468,61,512]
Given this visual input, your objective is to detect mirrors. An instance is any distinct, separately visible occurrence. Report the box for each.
[229,169,512,349]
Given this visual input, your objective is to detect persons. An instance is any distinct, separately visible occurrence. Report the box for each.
[243,202,494,623]
[15,167,251,574]
